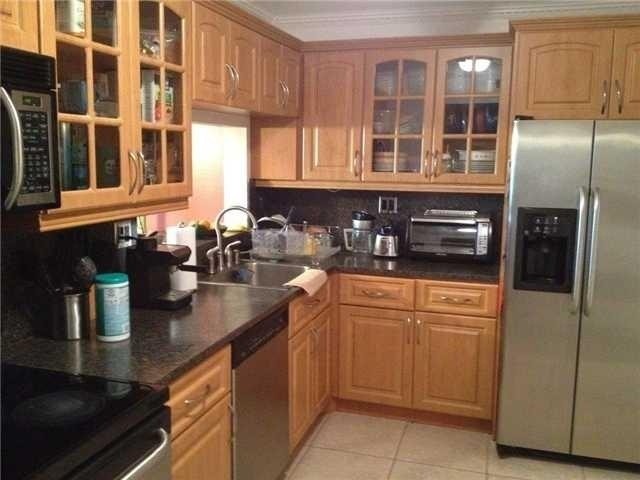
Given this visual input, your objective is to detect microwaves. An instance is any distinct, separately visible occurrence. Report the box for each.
[0,85,60,214]
[404,208,495,264]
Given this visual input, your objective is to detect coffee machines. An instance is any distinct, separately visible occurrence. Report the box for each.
[122,235,193,310]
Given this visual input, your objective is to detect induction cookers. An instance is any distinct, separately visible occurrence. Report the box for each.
[0,362,170,480]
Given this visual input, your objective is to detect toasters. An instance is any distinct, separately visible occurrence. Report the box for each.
[371,224,404,257]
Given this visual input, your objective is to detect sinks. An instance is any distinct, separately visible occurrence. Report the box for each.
[197,257,312,292]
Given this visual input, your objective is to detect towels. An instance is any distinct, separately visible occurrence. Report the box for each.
[284,268,328,297]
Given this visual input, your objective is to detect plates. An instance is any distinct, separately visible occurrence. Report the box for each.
[453,160,495,174]
[375,151,409,172]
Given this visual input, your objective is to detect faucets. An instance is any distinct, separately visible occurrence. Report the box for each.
[214,205,258,273]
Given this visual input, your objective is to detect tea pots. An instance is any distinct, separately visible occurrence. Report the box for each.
[343,209,375,254]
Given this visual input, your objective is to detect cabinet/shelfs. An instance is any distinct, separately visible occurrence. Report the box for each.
[345,32,513,194]
[507,12,639,118]
[0,1,192,235]
[253,41,362,190]
[287,272,337,458]
[180,0,260,114]
[248,17,304,120]
[331,274,500,437]
[164,343,234,480]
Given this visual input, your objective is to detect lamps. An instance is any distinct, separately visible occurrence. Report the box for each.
[459,58,491,73]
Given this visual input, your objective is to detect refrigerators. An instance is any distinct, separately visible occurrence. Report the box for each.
[494,117,639,473]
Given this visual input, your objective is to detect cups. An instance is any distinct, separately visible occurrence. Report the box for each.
[48,288,90,340]
